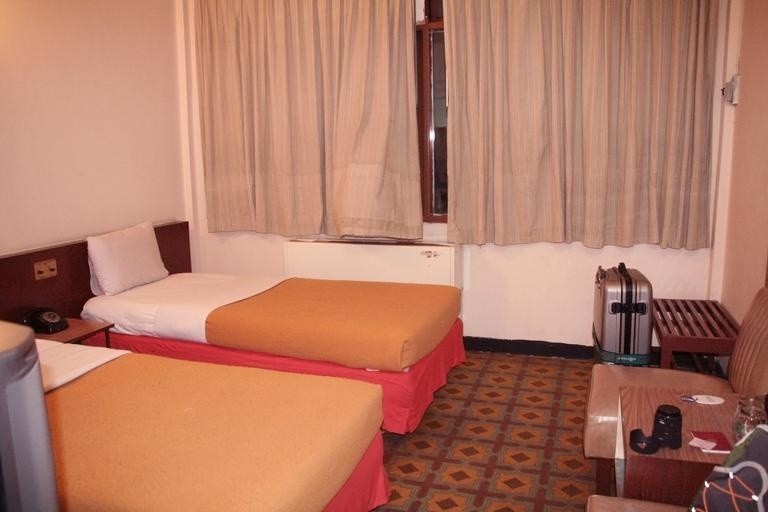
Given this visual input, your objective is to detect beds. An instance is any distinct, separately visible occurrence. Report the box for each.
[28,333,390,512]
[76,271,466,440]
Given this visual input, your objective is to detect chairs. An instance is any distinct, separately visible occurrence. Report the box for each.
[585,493,703,512]
[583,288,767,498]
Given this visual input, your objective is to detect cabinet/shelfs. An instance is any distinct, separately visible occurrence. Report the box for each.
[651,295,741,379]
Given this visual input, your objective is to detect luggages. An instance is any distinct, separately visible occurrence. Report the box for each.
[586,257,658,371]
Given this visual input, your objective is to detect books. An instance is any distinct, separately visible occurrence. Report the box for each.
[692,431,733,454]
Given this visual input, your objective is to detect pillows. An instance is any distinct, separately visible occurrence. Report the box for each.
[86,221,169,297]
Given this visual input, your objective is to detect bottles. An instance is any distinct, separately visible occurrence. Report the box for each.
[734,396,765,443]
[651,405,682,449]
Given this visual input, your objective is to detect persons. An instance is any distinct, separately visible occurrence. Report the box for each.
[691,395,768,512]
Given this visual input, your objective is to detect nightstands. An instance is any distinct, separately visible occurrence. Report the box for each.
[34,319,116,349]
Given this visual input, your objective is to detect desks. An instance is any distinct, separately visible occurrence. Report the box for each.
[612,382,746,506]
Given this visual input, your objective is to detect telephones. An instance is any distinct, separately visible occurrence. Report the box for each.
[21,307,69,335]
